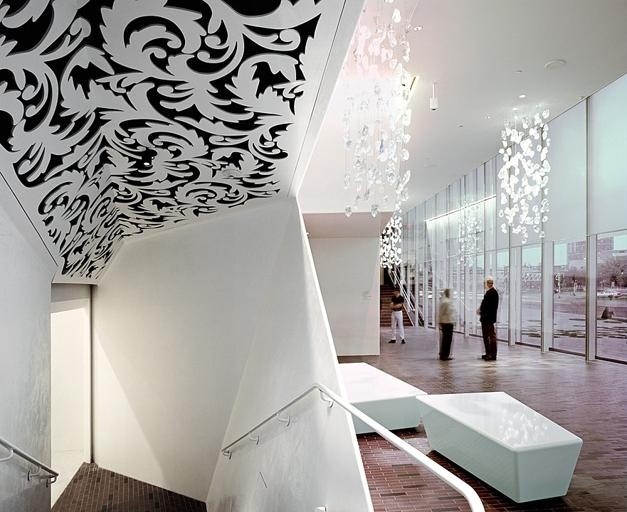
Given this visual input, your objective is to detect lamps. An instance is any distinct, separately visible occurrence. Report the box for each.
[379,216,403,269]
[498,107,551,244]
[341,9,413,216]
[454,198,482,271]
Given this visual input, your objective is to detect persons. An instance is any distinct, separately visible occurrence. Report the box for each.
[438,289,455,361]
[388,288,406,344]
[475,279,499,360]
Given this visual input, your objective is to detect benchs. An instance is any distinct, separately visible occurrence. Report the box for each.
[339,362,426,435]
[416,390,583,503]
